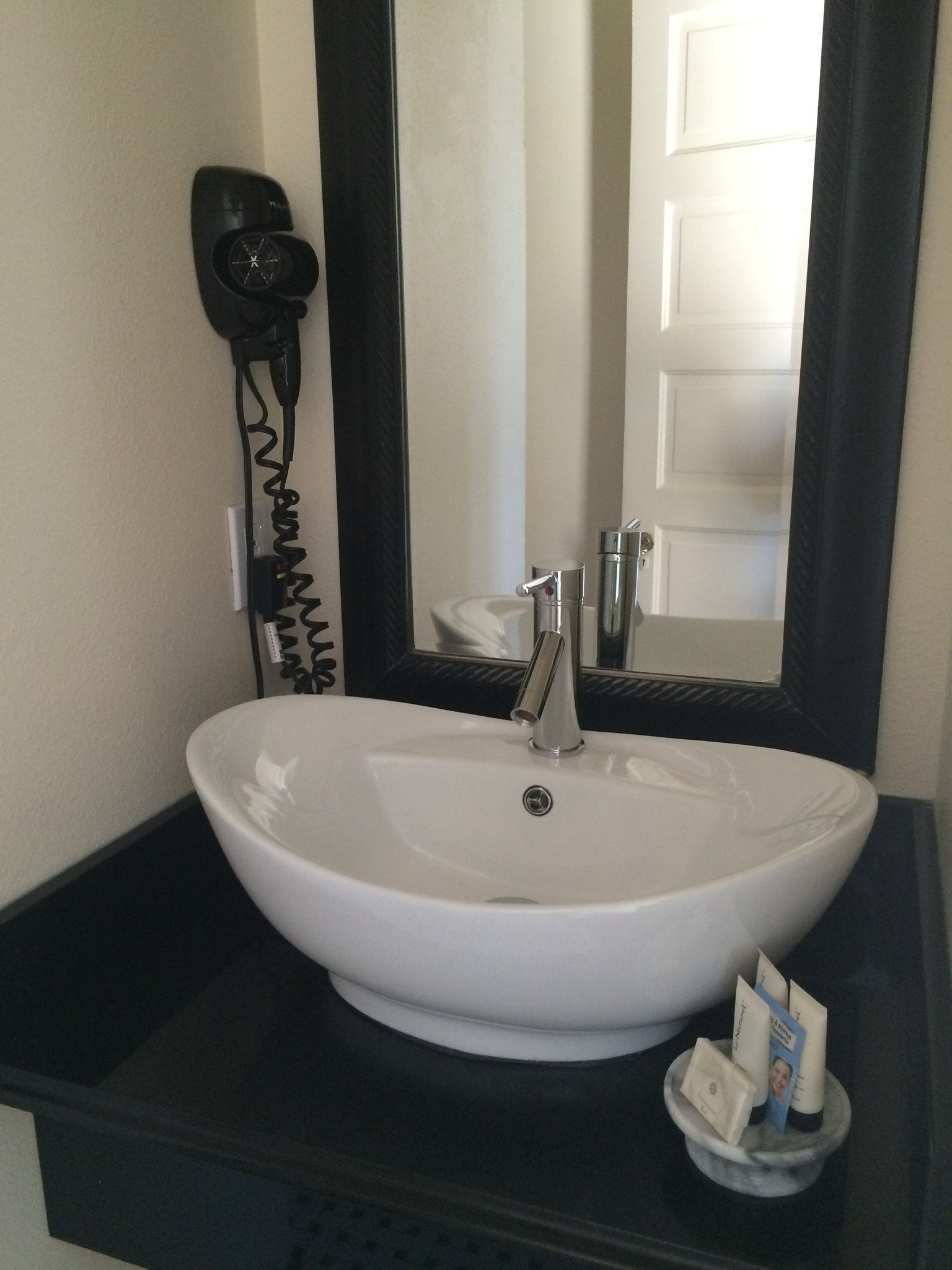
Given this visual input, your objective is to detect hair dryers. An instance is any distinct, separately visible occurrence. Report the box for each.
[230,230,339,414]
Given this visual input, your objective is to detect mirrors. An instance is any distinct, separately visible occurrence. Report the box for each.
[313,0,938,785]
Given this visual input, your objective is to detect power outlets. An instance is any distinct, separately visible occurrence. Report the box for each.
[228,500,270,613]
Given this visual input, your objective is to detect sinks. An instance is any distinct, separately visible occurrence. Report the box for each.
[183,693,879,1063]
[429,593,783,690]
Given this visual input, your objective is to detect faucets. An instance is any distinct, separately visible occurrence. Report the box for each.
[509,560,589,761]
[598,518,655,671]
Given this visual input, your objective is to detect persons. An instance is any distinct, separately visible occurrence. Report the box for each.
[769,1054,793,1103]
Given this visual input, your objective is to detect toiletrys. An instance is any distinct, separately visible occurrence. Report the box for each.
[730,973,770,1124]
[748,983,804,1132]
[751,944,789,1010]
[783,979,828,1131]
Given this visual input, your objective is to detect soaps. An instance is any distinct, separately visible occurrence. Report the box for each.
[680,1036,757,1147]
